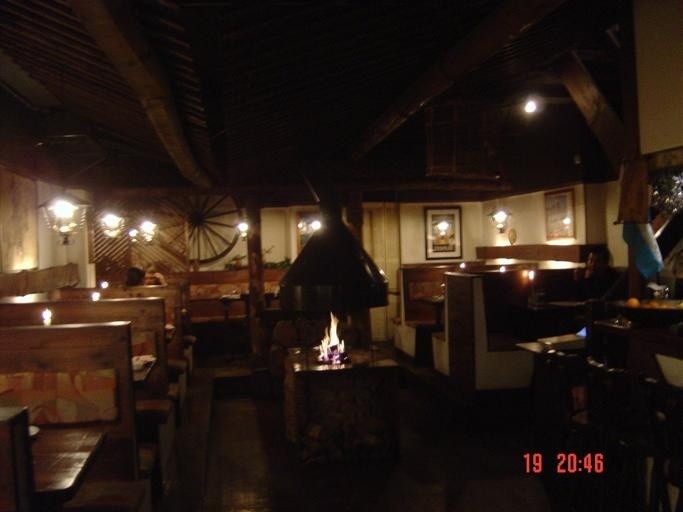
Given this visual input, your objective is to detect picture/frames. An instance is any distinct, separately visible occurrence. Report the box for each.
[425,206,464,259]
[546,188,575,240]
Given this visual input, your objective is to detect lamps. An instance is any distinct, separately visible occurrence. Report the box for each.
[312,220,321,231]
[493,210,507,234]
[98,210,127,242]
[39,184,95,244]
[238,223,249,240]
[139,219,159,242]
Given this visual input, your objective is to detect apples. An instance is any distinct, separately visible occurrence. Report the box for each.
[626,298,683,308]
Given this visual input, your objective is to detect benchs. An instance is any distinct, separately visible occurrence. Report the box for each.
[0,280,189,512]
[388,257,627,391]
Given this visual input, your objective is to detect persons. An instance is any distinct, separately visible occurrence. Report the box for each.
[125,267,146,287]
[569,247,627,302]
